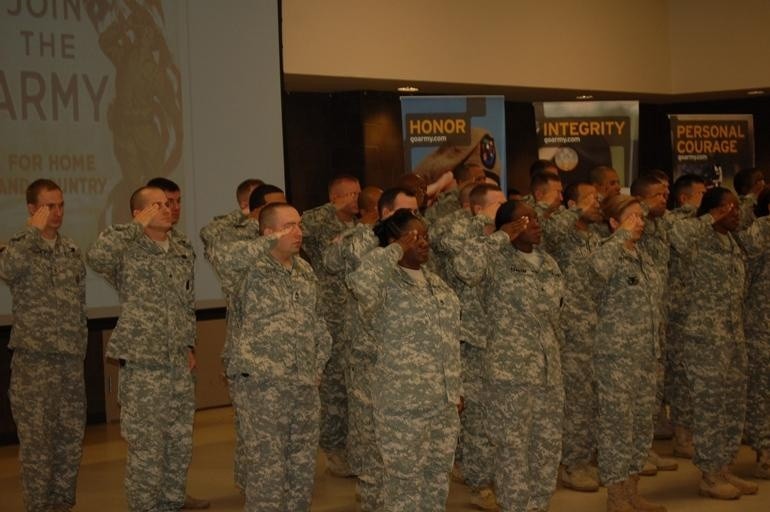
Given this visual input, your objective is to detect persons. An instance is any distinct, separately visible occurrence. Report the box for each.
[583,194,667,512]
[587,163,620,198]
[324,185,419,512]
[87,187,212,512]
[214,200,332,512]
[631,177,674,440]
[0,179,88,512]
[200,178,266,243]
[738,187,770,479]
[524,158,558,205]
[668,174,706,460]
[440,183,505,512]
[430,183,482,251]
[670,186,759,499]
[317,187,388,476]
[345,207,461,512]
[734,167,763,198]
[528,170,563,219]
[509,187,522,201]
[542,180,604,492]
[209,185,286,491]
[456,200,565,512]
[148,177,197,265]
[426,164,486,224]
[302,176,361,479]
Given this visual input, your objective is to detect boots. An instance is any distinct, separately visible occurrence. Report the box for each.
[605,481,637,512]
[467,488,503,511]
[324,453,357,478]
[450,460,467,485]
[181,492,210,511]
[752,447,770,479]
[720,463,759,494]
[653,404,673,440]
[587,464,606,488]
[626,475,668,512]
[639,461,658,475]
[561,465,600,493]
[672,439,696,459]
[648,449,679,471]
[697,471,741,500]
